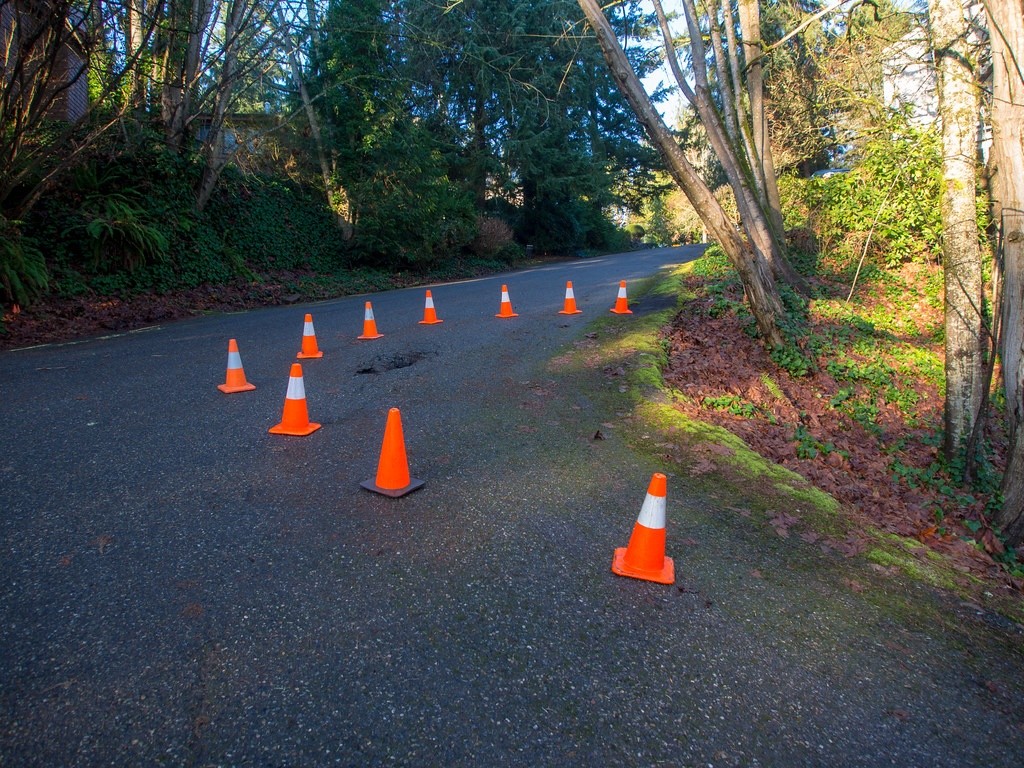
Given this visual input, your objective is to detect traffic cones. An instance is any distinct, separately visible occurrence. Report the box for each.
[495,285,519,318]
[216,339,256,393]
[418,290,444,324]
[267,363,321,436]
[296,313,324,358]
[558,281,582,315]
[611,472,676,585]
[356,302,384,339]
[359,406,426,498]
[609,280,634,315]
